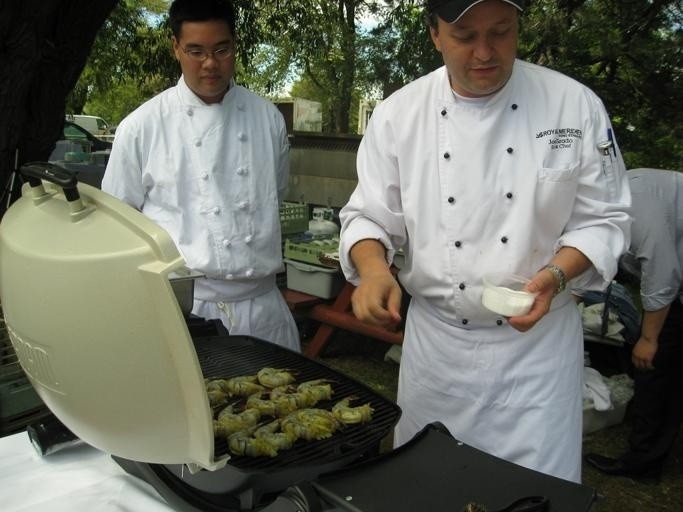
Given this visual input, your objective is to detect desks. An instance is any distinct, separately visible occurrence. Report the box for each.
[302,252,404,360]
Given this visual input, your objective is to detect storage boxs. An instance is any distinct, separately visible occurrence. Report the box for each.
[583,400,627,436]
[283,257,338,299]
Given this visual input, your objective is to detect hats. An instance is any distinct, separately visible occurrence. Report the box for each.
[428,0,527,24]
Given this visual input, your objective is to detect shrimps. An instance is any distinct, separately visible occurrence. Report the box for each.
[201,366,375,458]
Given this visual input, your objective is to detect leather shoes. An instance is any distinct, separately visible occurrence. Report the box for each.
[585,453,661,486]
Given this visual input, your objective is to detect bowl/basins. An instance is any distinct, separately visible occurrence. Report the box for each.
[479,273,543,320]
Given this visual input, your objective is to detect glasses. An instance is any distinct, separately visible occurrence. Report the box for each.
[178,42,237,62]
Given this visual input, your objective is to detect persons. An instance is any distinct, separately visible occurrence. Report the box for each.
[99,1,301,356]
[339,1,635,484]
[572,165,683,486]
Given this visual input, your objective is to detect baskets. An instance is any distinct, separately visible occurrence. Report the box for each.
[279,201,309,234]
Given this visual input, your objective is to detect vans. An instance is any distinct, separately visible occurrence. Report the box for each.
[63,115,114,142]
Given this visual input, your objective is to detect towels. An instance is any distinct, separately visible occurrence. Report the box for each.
[583,366,614,412]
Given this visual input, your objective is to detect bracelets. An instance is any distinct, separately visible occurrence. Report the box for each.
[537,263,566,296]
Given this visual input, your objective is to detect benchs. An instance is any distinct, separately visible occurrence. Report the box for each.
[281,289,320,308]
